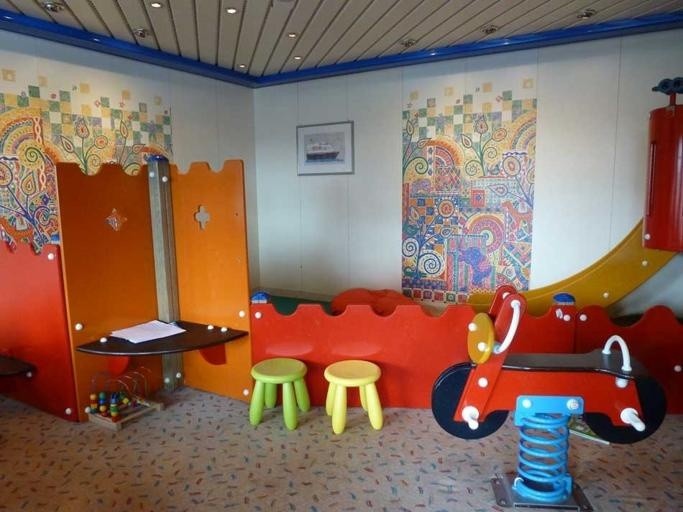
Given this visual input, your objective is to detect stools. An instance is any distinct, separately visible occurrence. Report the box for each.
[324,360,385,436]
[248,357,309,431]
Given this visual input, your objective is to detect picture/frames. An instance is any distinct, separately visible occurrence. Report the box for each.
[295,120,354,176]
